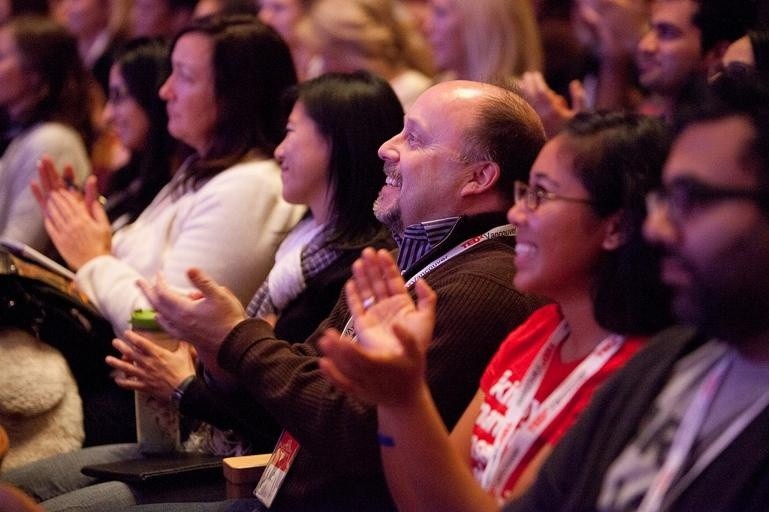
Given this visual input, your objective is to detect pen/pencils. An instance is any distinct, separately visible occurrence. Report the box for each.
[37,161,108,205]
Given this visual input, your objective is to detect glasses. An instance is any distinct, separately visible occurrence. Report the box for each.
[644,173,768,220]
[513,178,599,212]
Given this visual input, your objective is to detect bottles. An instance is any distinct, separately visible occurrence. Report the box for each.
[127,307,184,454]
